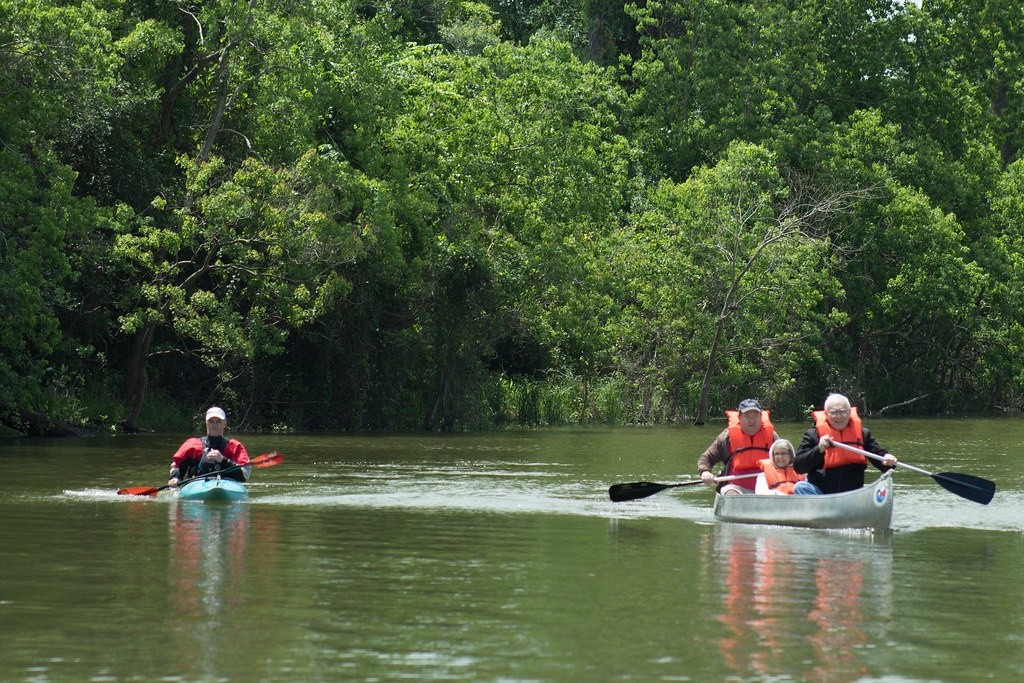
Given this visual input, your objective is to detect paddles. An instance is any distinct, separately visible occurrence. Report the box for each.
[117,450,284,497]
[609,470,766,502]
[825,440,997,505]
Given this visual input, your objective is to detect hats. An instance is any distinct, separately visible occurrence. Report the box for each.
[739,399,762,414]
[205,407,226,421]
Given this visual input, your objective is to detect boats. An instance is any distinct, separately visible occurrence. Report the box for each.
[709,467,895,533]
[170,471,249,503]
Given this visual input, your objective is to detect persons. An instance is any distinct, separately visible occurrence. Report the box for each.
[755,438,808,496]
[793,393,898,495]
[168,407,252,488]
[697,399,780,495]
